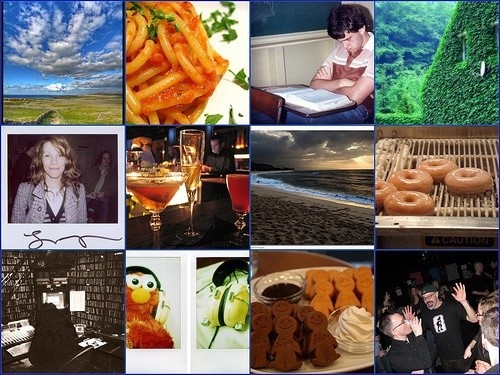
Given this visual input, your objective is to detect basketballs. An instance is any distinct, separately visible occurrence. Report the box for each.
[251,301,341,371]
[306,266,374,318]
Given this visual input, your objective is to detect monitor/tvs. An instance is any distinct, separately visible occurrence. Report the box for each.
[264,87,357,113]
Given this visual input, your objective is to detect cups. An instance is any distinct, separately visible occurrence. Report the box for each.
[475,311,484,317]
[393,318,406,331]
[423,293,435,300]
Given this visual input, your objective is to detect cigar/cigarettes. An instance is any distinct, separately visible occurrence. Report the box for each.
[125,1,229,124]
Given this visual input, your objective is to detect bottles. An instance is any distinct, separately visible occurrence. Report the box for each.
[421,284,437,294]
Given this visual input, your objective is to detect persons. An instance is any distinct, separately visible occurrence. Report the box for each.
[463,297,500,366]
[167,144,179,162]
[402,282,478,373]
[10,135,88,223]
[202,134,233,174]
[79,149,117,204]
[468,262,493,301]
[431,279,449,301]
[28,303,80,368]
[379,305,438,372]
[394,286,405,297]
[13,140,38,192]
[286,4,374,124]
[410,277,419,288]
[140,143,157,164]
[376,335,393,372]
[481,308,500,375]
[484,259,500,297]
[408,286,427,313]
[377,290,400,319]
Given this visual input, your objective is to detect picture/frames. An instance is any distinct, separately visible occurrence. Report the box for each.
[256,84,358,119]
[1,338,31,366]
[81,335,122,372]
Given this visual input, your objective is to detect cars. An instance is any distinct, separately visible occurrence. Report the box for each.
[404,314,419,327]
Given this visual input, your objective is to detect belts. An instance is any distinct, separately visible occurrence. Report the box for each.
[176,129,205,241]
[127,172,189,250]
[226,173,249,246]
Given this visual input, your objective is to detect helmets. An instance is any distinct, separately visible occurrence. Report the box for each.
[251,267,374,374]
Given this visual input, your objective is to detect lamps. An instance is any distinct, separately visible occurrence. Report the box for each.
[250,86,286,124]
[56,345,97,372]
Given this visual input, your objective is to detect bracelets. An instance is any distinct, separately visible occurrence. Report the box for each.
[254,272,306,304]
[328,304,374,355]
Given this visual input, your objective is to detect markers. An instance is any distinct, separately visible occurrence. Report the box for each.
[326,303,375,355]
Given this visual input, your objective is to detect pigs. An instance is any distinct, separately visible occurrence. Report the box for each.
[384,191,436,216]
[417,158,459,183]
[376,182,398,210]
[389,169,434,194]
[445,167,493,195]
